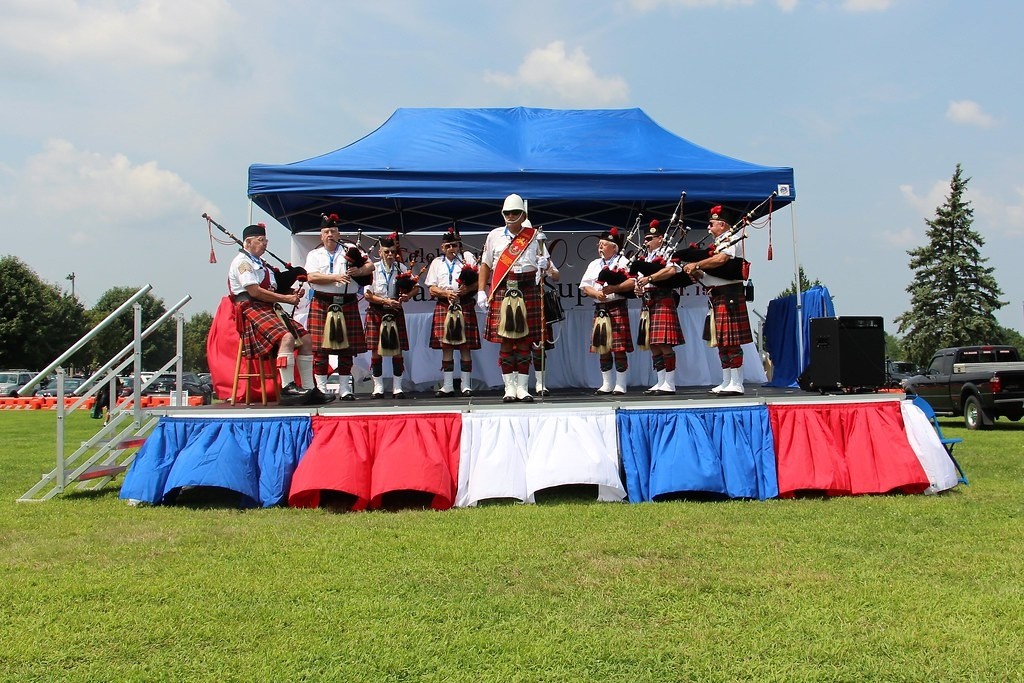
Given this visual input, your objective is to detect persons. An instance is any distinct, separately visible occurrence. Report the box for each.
[103,372,122,428]
[304,213,419,399]
[579,205,753,395]
[227,223,336,406]
[476,193,560,402]
[424,233,481,397]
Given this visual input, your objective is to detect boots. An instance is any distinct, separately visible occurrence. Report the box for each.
[370,375,384,399]
[612,369,628,395]
[502,371,550,402]
[276,353,355,406]
[597,370,612,395]
[644,368,676,396]
[707,365,744,396]
[392,373,405,399]
[434,371,472,398]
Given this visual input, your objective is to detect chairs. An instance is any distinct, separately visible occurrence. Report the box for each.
[905,395,969,486]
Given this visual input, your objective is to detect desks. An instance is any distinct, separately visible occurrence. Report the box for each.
[294,310,768,397]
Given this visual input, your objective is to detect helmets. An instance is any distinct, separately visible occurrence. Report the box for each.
[502,194,526,221]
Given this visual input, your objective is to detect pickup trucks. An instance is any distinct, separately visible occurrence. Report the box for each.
[902,345,1024,431]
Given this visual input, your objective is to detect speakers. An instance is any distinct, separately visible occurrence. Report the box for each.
[809,316,886,391]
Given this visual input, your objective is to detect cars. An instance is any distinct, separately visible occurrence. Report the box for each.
[325,372,354,394]
[0,369,216,406]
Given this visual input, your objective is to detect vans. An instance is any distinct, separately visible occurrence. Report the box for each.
[884,361,921,390]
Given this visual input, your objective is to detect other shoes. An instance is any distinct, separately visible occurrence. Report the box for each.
[103,422,107,427]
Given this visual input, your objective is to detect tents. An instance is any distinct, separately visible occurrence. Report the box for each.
[247,107,804,373]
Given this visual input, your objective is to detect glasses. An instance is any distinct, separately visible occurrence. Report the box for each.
[384,250,396,254]
[503,210,521,216]
[709,222,721,227]
[445,244,459,249]
[645,236,659,243]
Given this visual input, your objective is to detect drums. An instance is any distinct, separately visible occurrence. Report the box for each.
[544,289,567,325]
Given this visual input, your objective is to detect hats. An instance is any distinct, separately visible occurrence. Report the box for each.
[442,228,461,242]
[321,213,338,228]
[379,233,395,248]
[643,220,669,238]
[596,227,622,255]
[710,205,730,223]
[242,223,266,242]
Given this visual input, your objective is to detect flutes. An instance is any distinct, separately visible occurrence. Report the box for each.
[392,227,433,299]
[450,226,485,285]
[320,211,382,301]
[600,191,778,294]
[201,212,308,320]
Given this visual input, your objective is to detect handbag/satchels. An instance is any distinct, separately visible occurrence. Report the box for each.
[90,403,103,419]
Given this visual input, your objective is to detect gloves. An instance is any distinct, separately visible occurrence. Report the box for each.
[536,255,549,270]
[478,291,489,313]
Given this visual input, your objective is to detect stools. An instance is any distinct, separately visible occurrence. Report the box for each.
[231,337,280,406]
